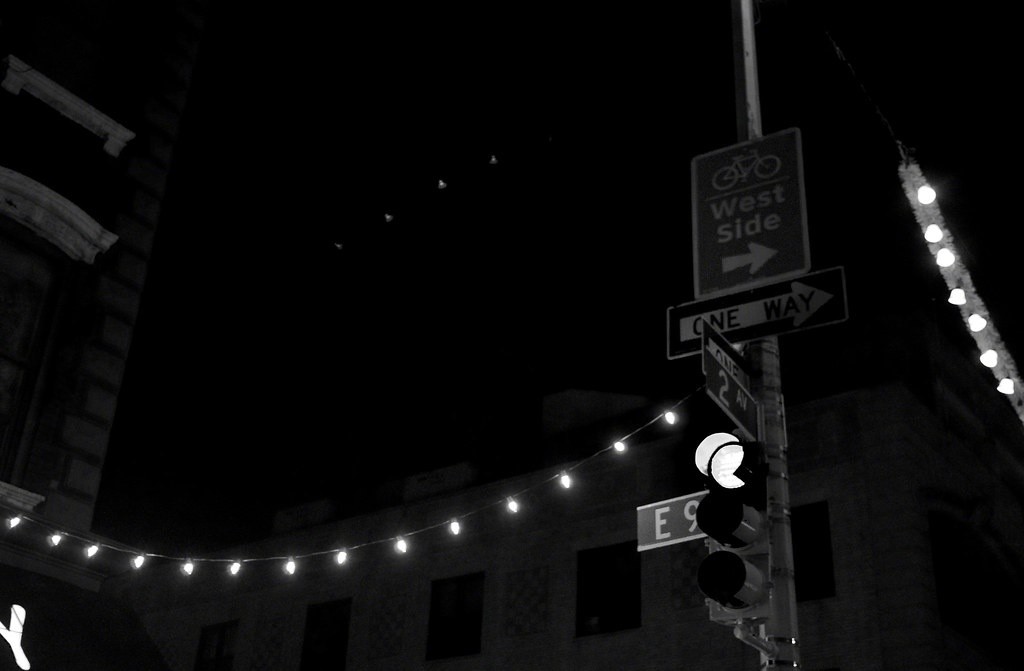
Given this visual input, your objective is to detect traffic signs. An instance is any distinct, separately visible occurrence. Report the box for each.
[664,266,851,362]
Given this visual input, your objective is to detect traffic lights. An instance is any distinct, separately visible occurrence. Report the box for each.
[691,426,777,628]
